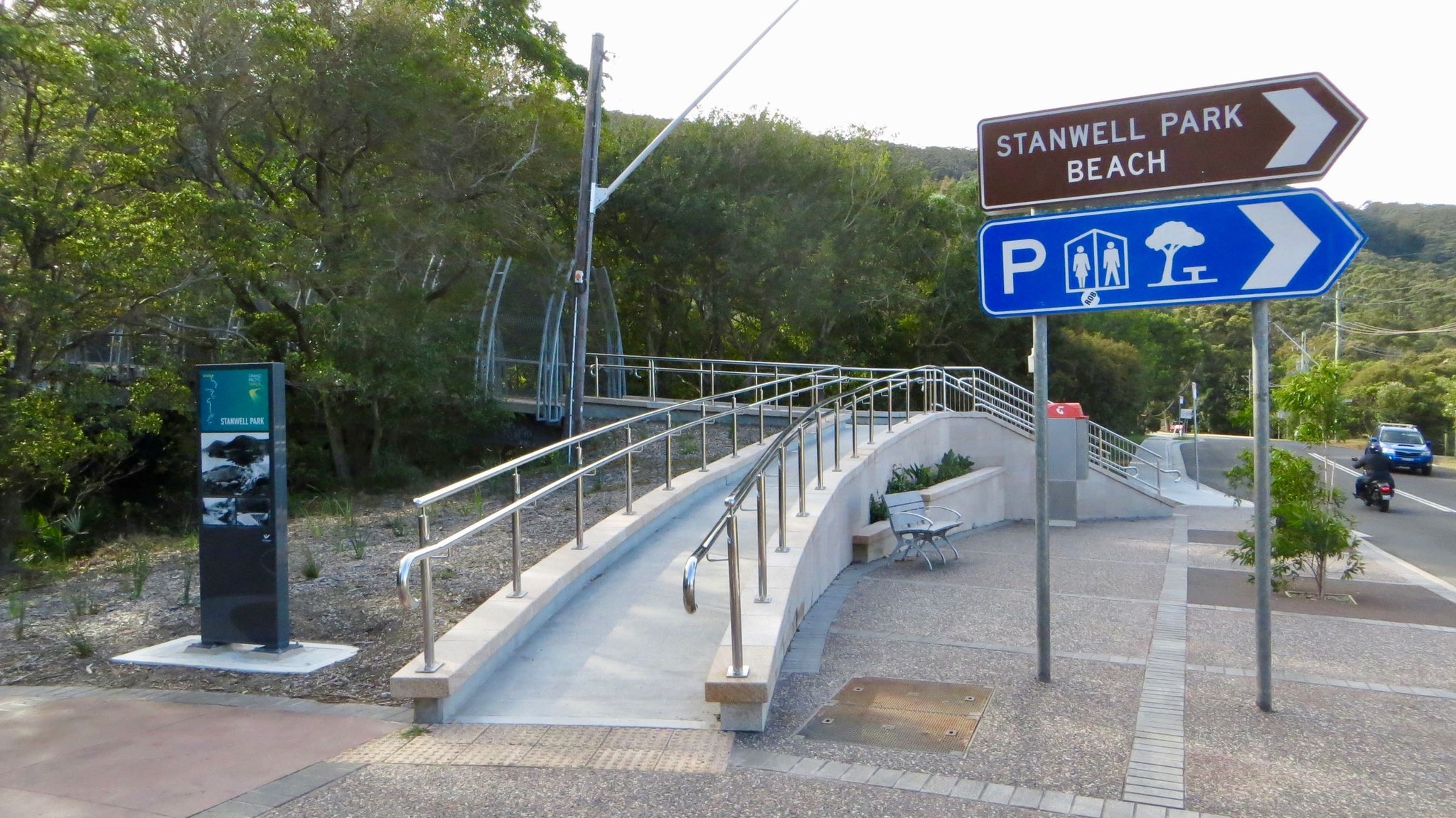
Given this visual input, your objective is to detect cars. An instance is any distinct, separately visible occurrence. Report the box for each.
[1169,420,1185,432]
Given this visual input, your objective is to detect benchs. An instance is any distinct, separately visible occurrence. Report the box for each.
[880,489,966,572]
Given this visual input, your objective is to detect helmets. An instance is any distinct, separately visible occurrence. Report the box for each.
[1370,445,1382,453]
[1371,437,1377,442]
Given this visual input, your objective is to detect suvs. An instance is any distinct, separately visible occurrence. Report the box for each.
[1367,421,1435,476]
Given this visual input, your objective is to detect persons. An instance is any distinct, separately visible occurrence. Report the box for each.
[1351,443,1395,498]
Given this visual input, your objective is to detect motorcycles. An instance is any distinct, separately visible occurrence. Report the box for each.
[1351,457,1394,512]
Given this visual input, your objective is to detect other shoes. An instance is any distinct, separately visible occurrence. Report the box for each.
[1353,493,1363,498]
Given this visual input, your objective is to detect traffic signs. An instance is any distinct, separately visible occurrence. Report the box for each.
[978,186,1367,319]
[976,72,1370,213]
